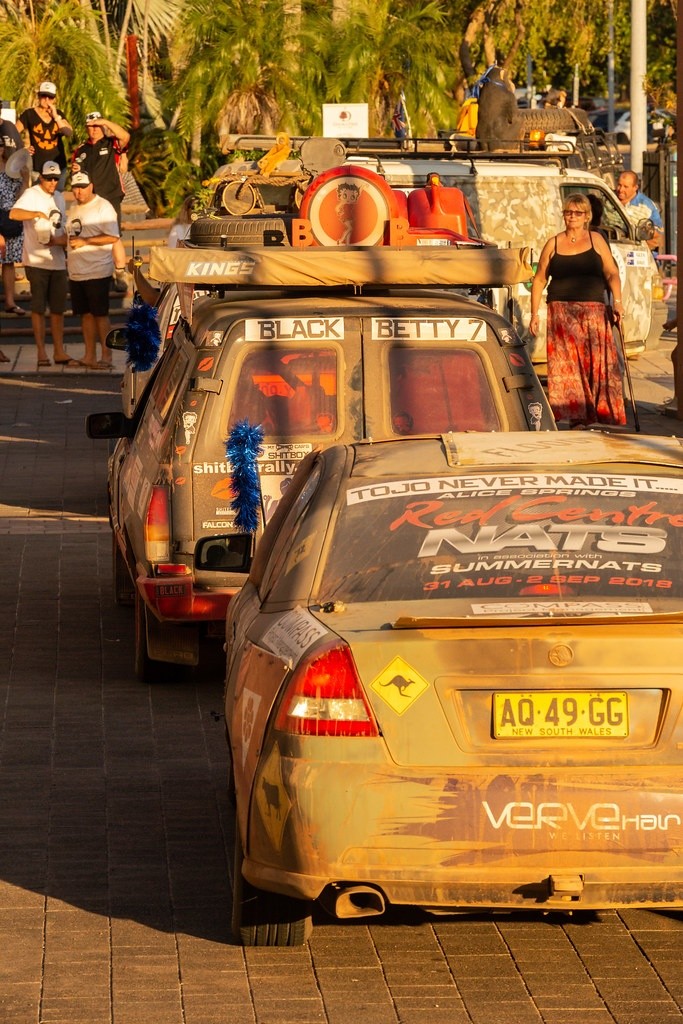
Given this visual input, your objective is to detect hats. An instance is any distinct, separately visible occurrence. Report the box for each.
[5,148,32,179]
[70,171,90,186]
[85,112,101,122]
[42,160,61,175]
[38,82,56,97]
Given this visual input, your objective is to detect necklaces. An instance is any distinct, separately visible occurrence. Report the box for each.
[571,238,575,242]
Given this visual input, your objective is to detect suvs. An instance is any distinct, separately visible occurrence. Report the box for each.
[83,214,564,685]
[179,126,672,391]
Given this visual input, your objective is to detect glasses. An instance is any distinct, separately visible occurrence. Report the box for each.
[562,210,585,216]
[44,177,59,181]
[89,115,103,120]
[41,95,54,98]
[0,144,5,147]
[189,206,195,210]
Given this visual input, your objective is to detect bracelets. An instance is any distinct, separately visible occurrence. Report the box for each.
[614,301,622,303]
[55,115,62,121]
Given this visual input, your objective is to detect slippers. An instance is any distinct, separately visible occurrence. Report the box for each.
[37,359,51,366]
[569,422,585,431]
[656,401,677,411]
[5,306,25,315]
[55,357,73,365]
[92,360,116,369]
[67,359,91,368]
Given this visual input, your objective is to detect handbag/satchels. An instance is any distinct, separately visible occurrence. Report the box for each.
[0,211,22,238]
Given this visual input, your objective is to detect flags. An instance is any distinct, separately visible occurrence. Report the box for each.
[392,101,405,142]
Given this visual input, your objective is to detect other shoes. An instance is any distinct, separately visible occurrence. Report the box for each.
[14,273,24,281]
[113,270,127,292]
[0,352,10,362]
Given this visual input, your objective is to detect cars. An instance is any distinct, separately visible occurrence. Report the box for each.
[192,425,682,955]
[586,106,677,146]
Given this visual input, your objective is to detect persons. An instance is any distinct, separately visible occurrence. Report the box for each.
[72,112,130,291]
[15,81,72,193]
[0,101,32,315]
[529,193,626,429]
[128,257,161,307]
[38,171,120,368]
[616,170,664,250]
[11,160,73,365]
